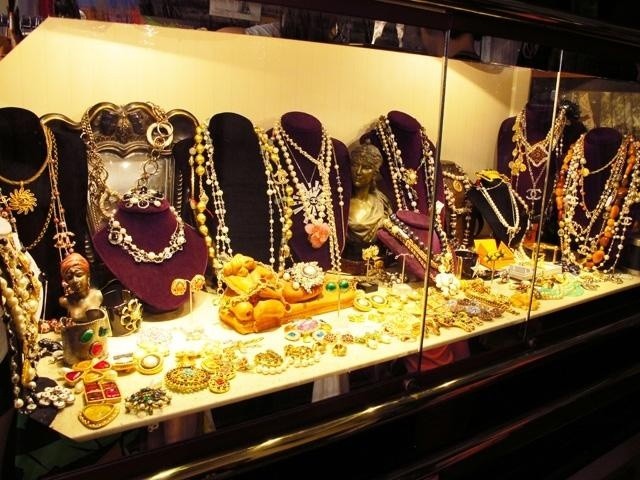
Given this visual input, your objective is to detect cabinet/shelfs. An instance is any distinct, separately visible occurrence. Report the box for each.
[0,0,640,480]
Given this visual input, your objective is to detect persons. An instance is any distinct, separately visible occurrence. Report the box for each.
[349,144,386,244]
[59,252,104,323]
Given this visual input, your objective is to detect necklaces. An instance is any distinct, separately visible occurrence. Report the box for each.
[272,112,347,276]
[464,289,521,317]
[106,205,187,266]
[436,162,474,254]
[0,122,77,300]
[81,97,175,220]
[383,213,463,299]
[472,179,522,248]
[189,120,294,279]
[164,364,210,394]
[555,128,640,271]
[0,233,48,418]
[507,100,571,245]
[374,114,436,215]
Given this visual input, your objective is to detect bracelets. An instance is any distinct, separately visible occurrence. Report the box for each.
[136,353,164,376]
[214,253,293,313]
[369,292,388,308]
[252,342,323,377]
[352,297,373,312]
[111,353,138,377]
[77,401,120,430]
